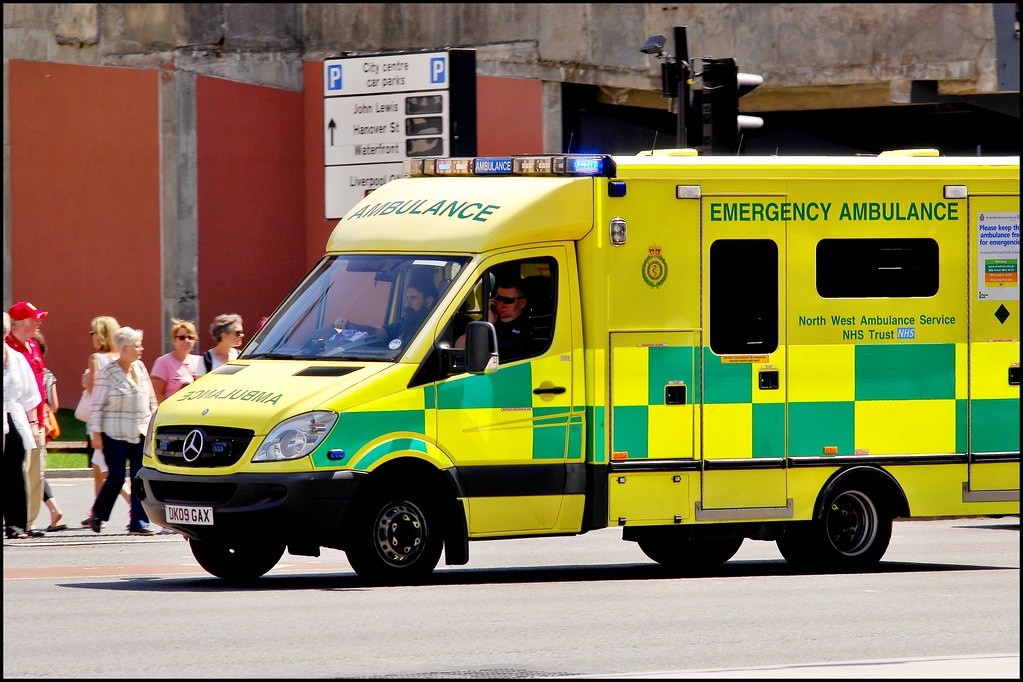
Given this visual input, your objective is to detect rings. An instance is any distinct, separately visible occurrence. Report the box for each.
[339,320,342,322]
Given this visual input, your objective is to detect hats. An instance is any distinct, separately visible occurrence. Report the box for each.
[9,302,48,321]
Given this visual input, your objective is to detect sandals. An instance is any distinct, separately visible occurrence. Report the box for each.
[8,532,28,539]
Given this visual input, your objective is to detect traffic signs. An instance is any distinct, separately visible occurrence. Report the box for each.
[323,91,451,166]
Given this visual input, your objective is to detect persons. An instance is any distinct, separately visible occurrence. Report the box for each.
[150,321,199,534]
[334,279,438,346]
[195,314,244,381]
[3,300,68,538]
[90,327,154,534]
[81,316,132,528]
[454,279,535,361]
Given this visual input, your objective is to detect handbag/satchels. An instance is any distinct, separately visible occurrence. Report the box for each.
[44,403,60,443]
[74,389,91,423]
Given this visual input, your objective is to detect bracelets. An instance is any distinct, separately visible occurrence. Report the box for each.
[29,420,39,423]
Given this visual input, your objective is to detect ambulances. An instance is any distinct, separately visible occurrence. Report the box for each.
[138,144,1020,582]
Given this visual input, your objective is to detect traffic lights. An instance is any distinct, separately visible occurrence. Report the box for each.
[704,56,764,155]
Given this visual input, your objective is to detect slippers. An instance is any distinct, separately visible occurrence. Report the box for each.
[46,525,66,531]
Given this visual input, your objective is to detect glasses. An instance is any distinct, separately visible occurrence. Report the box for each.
[495,294,523,304]
[89,331,95,336]
[225,330,243,337]
[125,343,143,348]
[174,335,195,340]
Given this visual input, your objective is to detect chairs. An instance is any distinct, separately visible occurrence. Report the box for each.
[525,275,551,352]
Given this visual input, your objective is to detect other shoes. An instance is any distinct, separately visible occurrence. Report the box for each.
[26,529,44,537]
[81,510,91,527]
[129,521,154,534]
[91,509,101,533]
